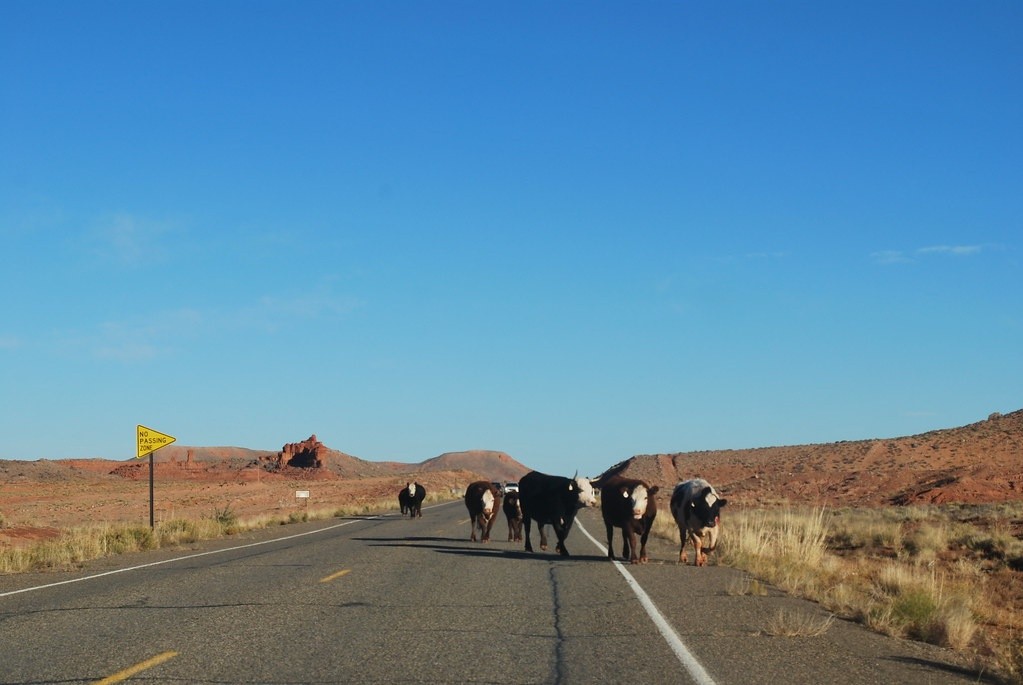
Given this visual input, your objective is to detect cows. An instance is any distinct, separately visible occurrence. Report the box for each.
[518,469,597,557]
[465,480,501,544]
[398,481,426,519]
[502,489,524,543]
[599,475,660,565]
[670,478,727,567]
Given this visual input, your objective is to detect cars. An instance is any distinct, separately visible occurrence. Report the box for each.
[503,481,519,494]
